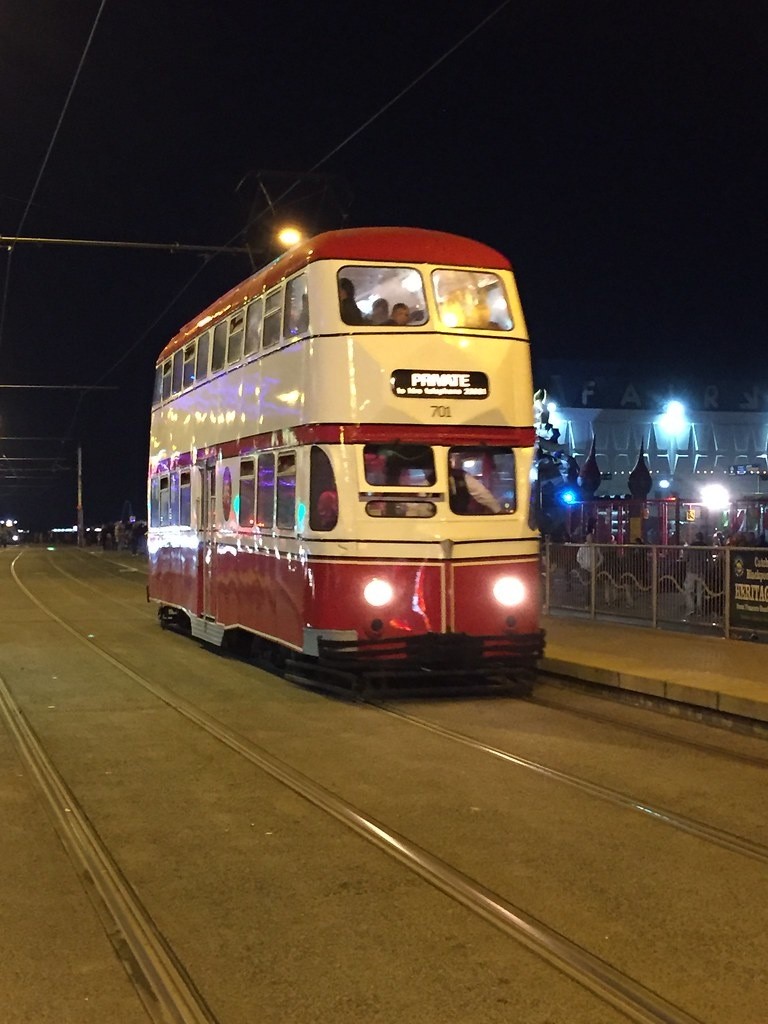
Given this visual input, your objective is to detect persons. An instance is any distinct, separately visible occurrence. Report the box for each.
[338,277,366,326]
[100,519,148,560]
[381,304,424,328]
[410,456,513,515]
[362,298,388,327]
[554,514,768,621]
[297,295,308,333]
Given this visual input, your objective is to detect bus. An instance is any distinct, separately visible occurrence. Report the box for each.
[144,227,545,695]
